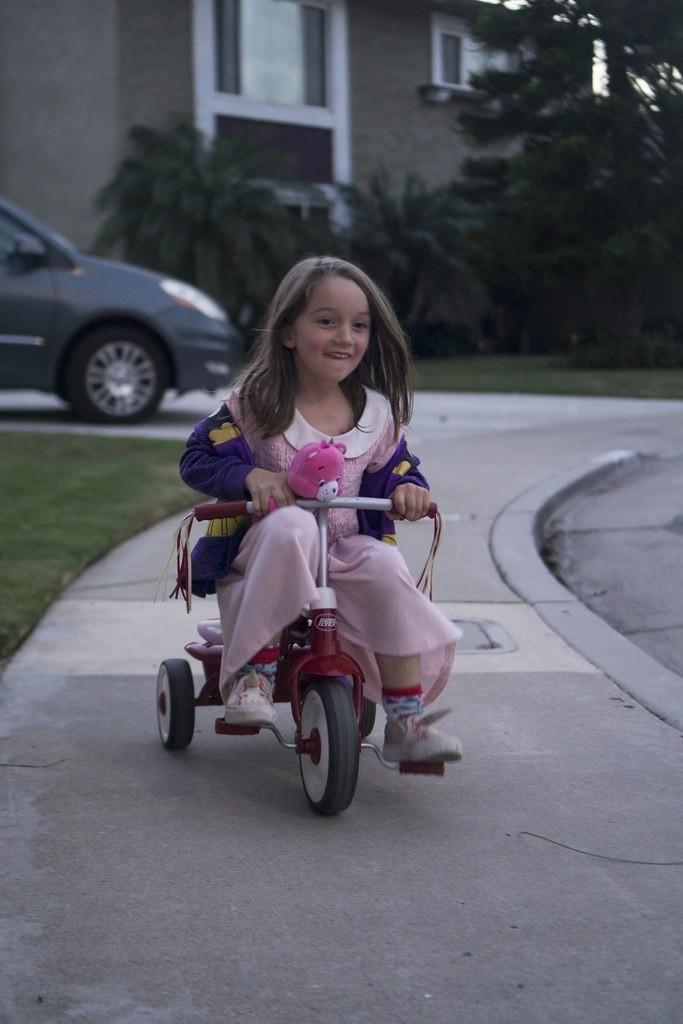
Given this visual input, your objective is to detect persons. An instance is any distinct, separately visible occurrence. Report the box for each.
[179,257,464,767]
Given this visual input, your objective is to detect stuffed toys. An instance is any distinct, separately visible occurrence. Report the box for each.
[251,439,346,526]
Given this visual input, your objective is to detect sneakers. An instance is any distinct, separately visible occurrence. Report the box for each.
[225,670,276,726]
[382,708,463,763]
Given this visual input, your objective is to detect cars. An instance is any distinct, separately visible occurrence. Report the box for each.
[0,199,244,425]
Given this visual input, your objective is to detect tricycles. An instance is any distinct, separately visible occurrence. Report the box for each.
[156,496,444,816]
